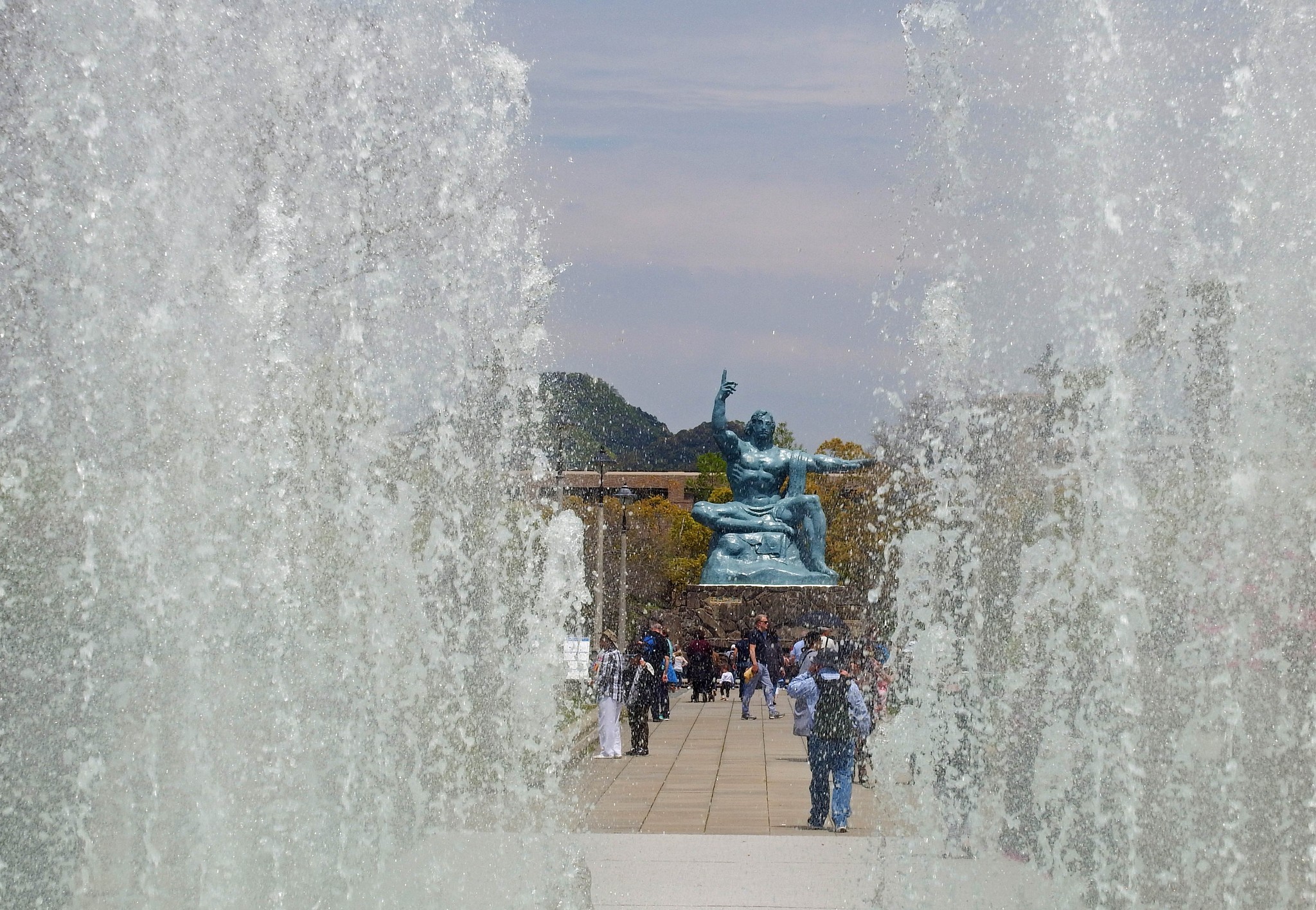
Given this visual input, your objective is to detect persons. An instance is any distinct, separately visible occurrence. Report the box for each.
[688,614,896,832]
[593,629,625,759]
[691,367,876,575]
[625,623,688,756]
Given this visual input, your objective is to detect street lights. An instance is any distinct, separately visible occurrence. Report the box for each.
[586,444,616,653]
[613,480,638,655]
[1024,341,1070,502]
[550,402,580,516]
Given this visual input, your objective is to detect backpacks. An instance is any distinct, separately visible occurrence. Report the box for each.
[814,670,852,739]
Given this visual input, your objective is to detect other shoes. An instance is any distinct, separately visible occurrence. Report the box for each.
[663,715,670,720]
[836,826,848,834]
[625,749,649,756]
[652,718,663,722]
[720,695,728,701]
[595,753,622,758]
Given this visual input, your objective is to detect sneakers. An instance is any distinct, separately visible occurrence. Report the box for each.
[741,715,756,720]
[769,712,785,718]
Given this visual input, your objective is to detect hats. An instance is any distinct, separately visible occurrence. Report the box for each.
[602,628,619,648]
[813,648,839,667]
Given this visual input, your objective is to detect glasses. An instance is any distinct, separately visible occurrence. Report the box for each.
[761,621,769,625]
